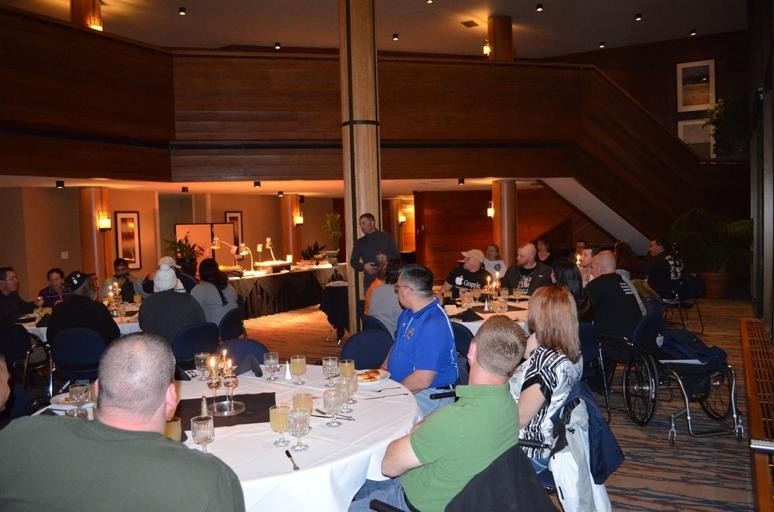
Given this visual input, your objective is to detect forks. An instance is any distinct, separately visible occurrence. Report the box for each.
[368,386,401,393]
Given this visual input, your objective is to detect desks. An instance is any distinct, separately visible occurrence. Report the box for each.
[226,262,347,320]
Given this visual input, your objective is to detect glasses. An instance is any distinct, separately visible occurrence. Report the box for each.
[394,283,413,293]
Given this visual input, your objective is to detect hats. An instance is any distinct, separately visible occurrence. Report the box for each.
[65,271,98,292]
[158,256,182,269]
[460,248,486,264]
[151,264,178,293]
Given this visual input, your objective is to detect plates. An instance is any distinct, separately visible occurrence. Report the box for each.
[356,368,391,383]
[48,392,95,408]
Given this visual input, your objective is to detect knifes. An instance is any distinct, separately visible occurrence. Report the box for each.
[286,451,300,471]
[362,392,413,400]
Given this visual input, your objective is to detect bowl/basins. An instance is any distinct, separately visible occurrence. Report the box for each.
[296,251,340,268]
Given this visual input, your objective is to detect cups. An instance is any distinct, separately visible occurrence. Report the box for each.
[67,383,95,422]
[165,417,183,441]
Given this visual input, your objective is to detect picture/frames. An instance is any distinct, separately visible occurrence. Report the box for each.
[115,211,142,270]
[677,59,716,112]
[677,118,717,165]
[225,211,244,261]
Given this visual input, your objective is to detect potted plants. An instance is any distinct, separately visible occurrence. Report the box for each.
[162,232,204,277]
[665,209,754,299]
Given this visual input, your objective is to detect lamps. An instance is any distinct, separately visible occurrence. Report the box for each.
[210,236,280,278]
[295,216,304,229]
[98,218,112,232]
[399,216,407,226]
[486,201,494,219]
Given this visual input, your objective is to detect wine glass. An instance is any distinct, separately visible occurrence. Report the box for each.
[191,416,215,455]
[265,353,357,450]
[376,249,384,266]
[196,351,246,417]
[100,282,142,324]
[32,296,43,328]
[431,284,522,315]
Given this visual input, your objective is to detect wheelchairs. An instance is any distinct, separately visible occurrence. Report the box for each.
[623,325,748,445]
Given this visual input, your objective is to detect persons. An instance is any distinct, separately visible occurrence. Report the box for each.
[3,255,244,510]
[347,212,682,511]
